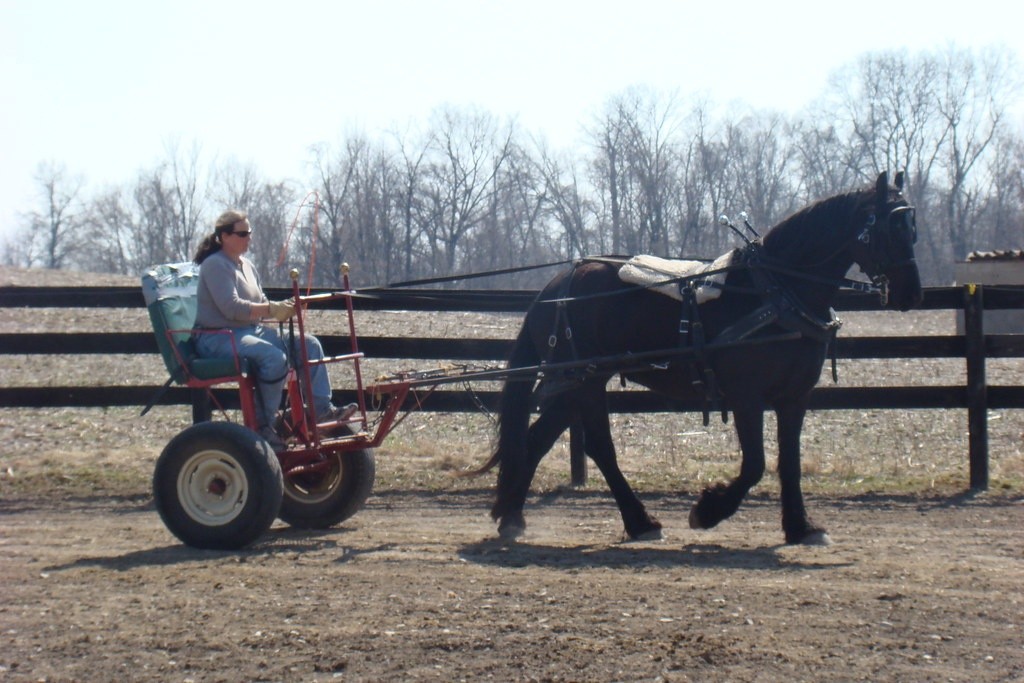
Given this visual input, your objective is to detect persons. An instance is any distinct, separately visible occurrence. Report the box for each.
[190,210,359,449]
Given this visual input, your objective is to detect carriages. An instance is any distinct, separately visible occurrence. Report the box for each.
[142,172,922,549]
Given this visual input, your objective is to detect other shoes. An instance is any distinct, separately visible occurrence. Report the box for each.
[315,403,359,435]
[263,430,287,451]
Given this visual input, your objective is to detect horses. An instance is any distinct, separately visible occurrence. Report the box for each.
[490,170,924,546]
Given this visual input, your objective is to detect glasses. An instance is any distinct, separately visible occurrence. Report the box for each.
[226,230,252,237]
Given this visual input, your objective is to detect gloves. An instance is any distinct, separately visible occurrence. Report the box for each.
[269,296,307,322]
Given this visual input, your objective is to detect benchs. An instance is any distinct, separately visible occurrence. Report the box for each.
[141,262,310,445]
